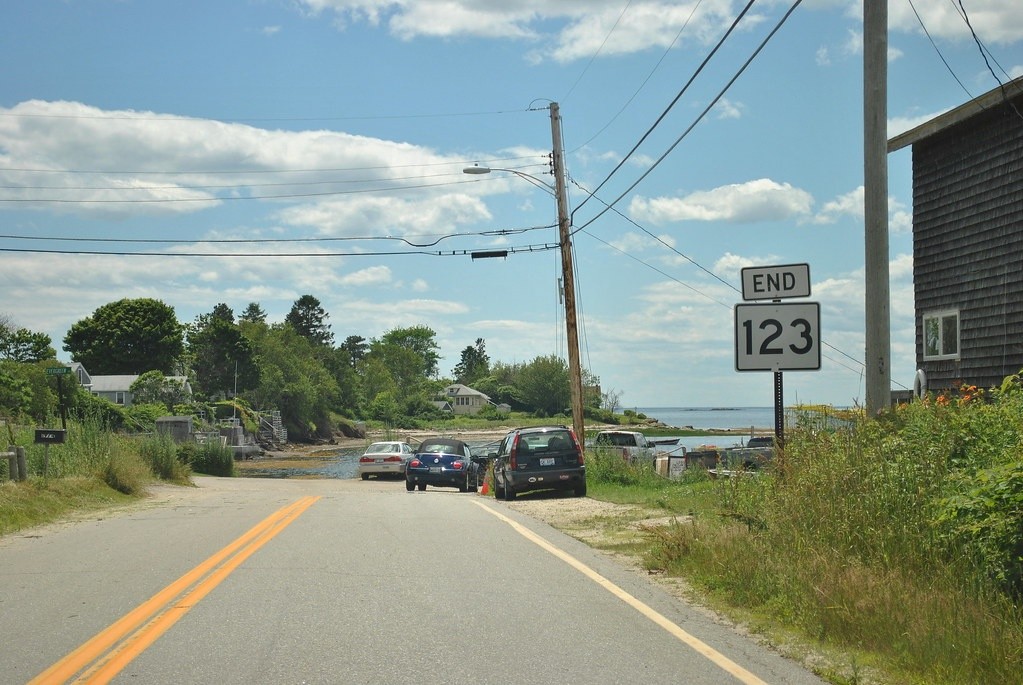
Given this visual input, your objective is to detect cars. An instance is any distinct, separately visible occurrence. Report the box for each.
[404,438,480,492]
[359,441,414,480]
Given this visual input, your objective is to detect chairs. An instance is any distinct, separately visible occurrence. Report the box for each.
[521,438,529,451]
[547,437,561,451]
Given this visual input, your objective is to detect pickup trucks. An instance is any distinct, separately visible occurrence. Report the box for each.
[586,430,657,470]
[729,434,775,473]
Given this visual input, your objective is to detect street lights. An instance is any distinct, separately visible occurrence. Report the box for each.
[463,103,585,464]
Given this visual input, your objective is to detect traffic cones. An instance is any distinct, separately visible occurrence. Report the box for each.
[480,469,490,495]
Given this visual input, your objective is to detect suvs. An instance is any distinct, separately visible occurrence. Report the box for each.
[487,425,587,502]
